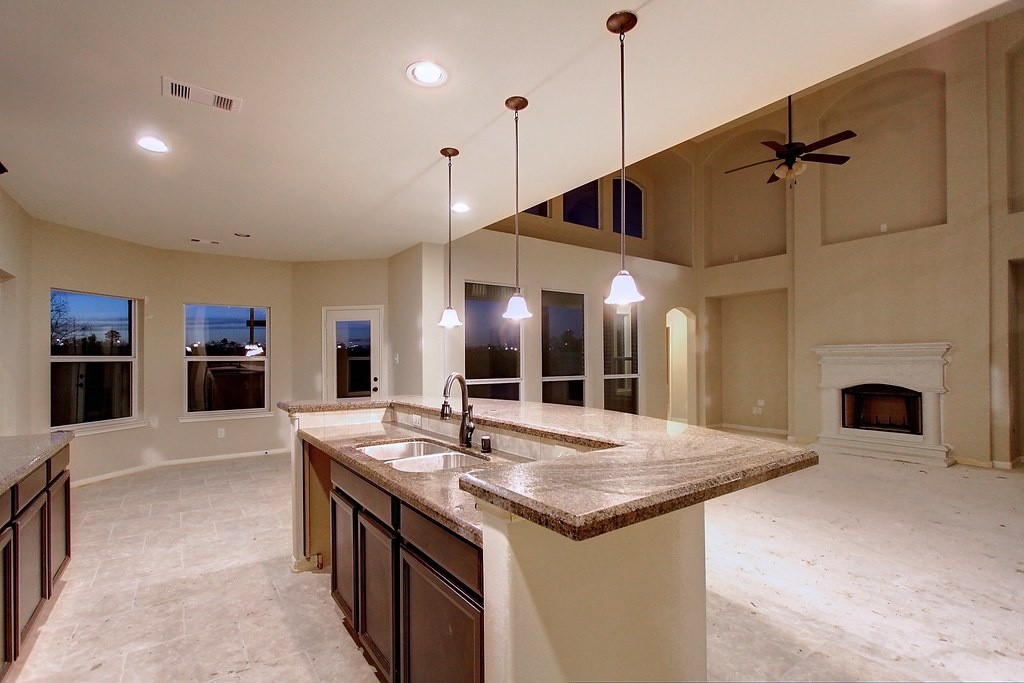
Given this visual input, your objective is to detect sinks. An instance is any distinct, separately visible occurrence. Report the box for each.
[356,443,452,462]
[385,450,491,474]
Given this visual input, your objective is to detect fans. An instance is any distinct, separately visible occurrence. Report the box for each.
[726,102,858,188]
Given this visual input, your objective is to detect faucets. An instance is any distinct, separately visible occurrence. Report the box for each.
[439,372,474,447]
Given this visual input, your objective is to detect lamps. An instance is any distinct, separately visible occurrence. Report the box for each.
[501,95,536,321]
[435,147,464,330]
[604,9,648,308]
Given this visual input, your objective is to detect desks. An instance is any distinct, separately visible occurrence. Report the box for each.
[279,390,820,681]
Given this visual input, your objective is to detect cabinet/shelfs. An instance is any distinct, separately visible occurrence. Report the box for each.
[0,431,76,683]
